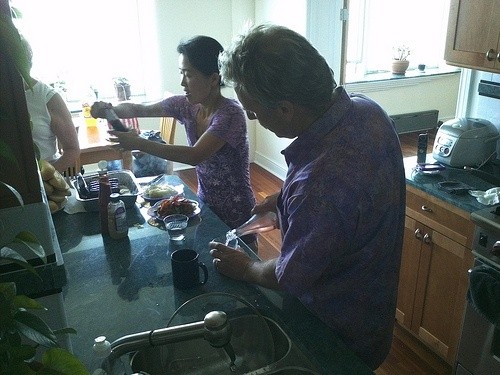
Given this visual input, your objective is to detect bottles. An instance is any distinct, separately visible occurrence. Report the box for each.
[227,208,279,241]
[89,335,126,375]
[106,192,128,240]
[97,160,112,235]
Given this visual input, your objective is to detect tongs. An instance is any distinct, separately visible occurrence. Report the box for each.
[75,172,89,191]
[138,174,166,196]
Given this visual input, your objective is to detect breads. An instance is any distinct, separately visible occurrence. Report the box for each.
[38,159,71,212]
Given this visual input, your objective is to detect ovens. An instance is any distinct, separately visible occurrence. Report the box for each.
[455,257,500,375]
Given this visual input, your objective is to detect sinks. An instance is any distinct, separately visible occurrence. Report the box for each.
[102,314,291,375]
[264,365,317,375]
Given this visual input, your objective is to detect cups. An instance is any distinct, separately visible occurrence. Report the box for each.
[163,213,189,241]
[170,248,208,291]
[211,235,239,250]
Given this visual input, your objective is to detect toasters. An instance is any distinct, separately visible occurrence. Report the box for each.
[432,117,500,168]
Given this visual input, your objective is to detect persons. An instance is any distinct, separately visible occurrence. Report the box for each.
[207,23,407,369]
[91,35,260,255]
[17,36,81,173]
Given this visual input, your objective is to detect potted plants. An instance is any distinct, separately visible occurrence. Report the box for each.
[389,45,414,74]
[113,76,131,100]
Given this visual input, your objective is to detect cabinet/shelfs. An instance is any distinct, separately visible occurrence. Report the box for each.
[395,184,473,369]
[443,0,500,74]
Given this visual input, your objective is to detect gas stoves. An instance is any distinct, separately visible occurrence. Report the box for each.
[470,203,500,270]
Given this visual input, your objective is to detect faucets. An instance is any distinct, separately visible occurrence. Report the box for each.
[91,309,234,375]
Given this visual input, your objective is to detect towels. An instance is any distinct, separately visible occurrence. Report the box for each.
[468,187,500,205]
[467,266,500,327]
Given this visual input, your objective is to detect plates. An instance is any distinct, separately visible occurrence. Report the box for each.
[141,186,178,201]
[153,198,202,222]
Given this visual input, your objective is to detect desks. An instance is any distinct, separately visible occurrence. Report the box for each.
[63,111,132,169]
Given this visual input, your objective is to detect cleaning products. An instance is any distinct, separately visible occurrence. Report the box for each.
[90,335,131,375]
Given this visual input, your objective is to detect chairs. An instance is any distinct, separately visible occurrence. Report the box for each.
[159,90,179,175]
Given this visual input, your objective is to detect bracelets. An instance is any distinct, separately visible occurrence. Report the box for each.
[162,145,175,158]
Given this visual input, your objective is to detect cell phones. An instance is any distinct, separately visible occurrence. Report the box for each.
[418,163,445,170]
